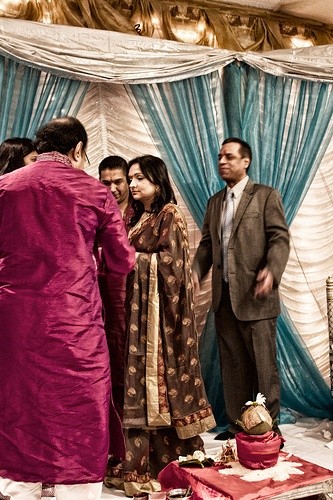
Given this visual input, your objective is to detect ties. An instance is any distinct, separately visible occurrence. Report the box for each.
[222,189,234,283]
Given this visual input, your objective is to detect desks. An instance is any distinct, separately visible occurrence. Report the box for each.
[158,452,333,500]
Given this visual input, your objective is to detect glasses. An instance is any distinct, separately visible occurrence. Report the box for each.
[82,147,91,168]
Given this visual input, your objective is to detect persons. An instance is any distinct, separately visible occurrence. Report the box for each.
[103,156,217,500]
[92,156,132,384]
[0,116,138,500]
[0,137,40,179]
[190,136,291,449]
